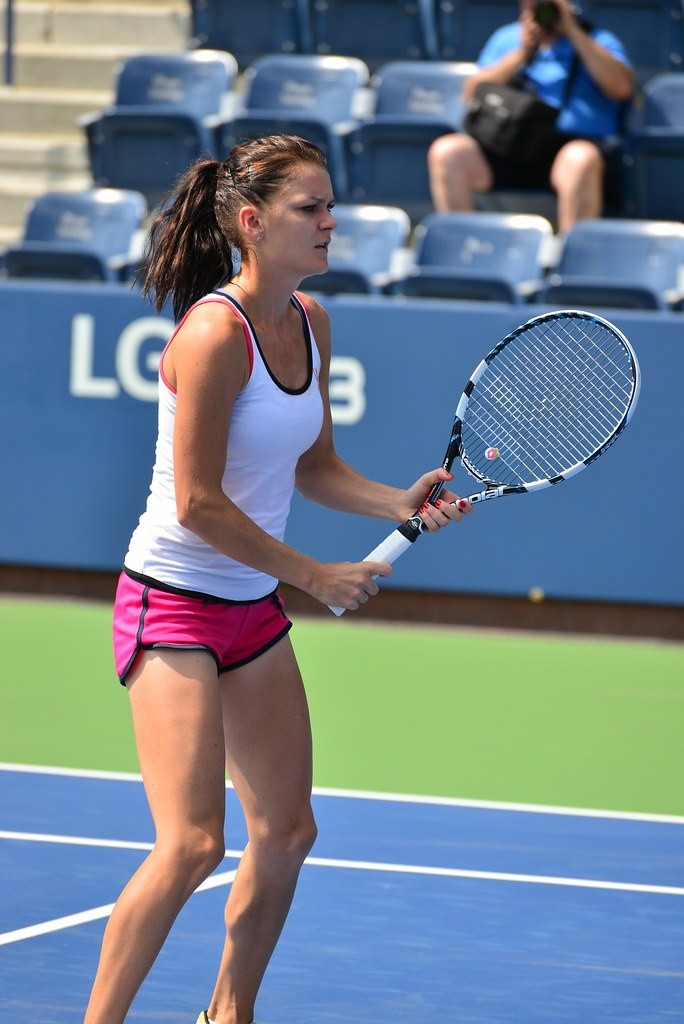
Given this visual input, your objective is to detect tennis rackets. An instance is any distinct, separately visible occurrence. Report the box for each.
[324,308,643,618]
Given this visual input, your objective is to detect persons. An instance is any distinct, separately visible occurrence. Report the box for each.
[81,133,471,1024]
[427,0,638,233]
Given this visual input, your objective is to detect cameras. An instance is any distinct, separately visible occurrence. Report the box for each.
[533,0,560,28]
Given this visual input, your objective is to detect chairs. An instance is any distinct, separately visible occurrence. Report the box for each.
[0,1,684,317]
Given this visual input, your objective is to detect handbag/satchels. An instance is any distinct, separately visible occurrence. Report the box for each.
[465,80,561,165]
